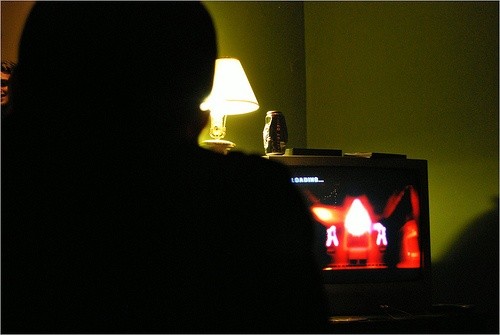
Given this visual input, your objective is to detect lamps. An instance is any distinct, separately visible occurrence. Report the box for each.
[196,57,260,152]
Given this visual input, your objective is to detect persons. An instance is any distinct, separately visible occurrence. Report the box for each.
[1,0,332,335]
[0,56,16,105]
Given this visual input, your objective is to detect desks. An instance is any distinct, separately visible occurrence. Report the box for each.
[325,301,476,334]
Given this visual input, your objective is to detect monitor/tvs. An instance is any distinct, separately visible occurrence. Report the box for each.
[262,155,433,284]
